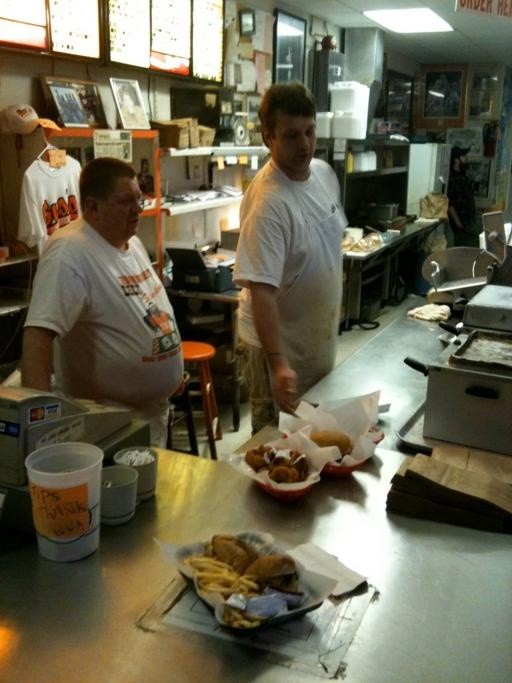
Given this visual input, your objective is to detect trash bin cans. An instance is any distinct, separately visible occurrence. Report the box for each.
[413,250,434,296]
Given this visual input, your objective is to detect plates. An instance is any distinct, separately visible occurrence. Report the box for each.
[174,530,324,633]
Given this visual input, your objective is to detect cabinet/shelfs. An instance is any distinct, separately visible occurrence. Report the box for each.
[0,128,163,377]
[345,139,410,179]
[160,146,271,218]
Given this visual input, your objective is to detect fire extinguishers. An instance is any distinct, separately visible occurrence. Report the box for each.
[483,120,499,157]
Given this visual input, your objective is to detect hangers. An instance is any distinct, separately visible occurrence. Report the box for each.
[35,129,67,164]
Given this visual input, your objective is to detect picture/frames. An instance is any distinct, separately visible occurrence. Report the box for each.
[252,49,272,93]
[40,73,108,128]
[0,0,225,87]
[108,77,152,129]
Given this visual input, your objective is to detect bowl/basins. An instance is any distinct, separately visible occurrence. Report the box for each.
[255,422,384,501]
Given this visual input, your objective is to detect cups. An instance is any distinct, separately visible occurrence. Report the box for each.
[101,465,139,525]
[113,446,158,501]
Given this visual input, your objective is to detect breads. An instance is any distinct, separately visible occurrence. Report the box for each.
[211,534,259,576]
[307,429,351,453]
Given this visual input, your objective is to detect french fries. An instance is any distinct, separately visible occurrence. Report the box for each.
[191,558,258,622]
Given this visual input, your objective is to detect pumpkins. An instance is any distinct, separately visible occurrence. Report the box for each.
[4,104,39,135]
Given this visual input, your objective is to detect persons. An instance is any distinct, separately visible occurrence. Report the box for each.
[447,145,481,247]
[229,80,346,434]
[16,156,188,449]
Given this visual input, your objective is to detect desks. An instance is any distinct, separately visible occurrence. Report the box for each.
[0,447,512,683]
[160,289,244,432]
[339,213,445,332]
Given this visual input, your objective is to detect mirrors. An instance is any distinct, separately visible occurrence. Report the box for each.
[272,8,308,87]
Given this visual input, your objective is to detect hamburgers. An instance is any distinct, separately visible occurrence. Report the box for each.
[244,555,297,596]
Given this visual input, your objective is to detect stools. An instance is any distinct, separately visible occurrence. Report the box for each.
[167,341,222,460]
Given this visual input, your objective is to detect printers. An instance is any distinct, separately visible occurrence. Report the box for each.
[166,247,235,292]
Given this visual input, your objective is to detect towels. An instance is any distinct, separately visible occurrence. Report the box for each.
[409,302,452,322]
